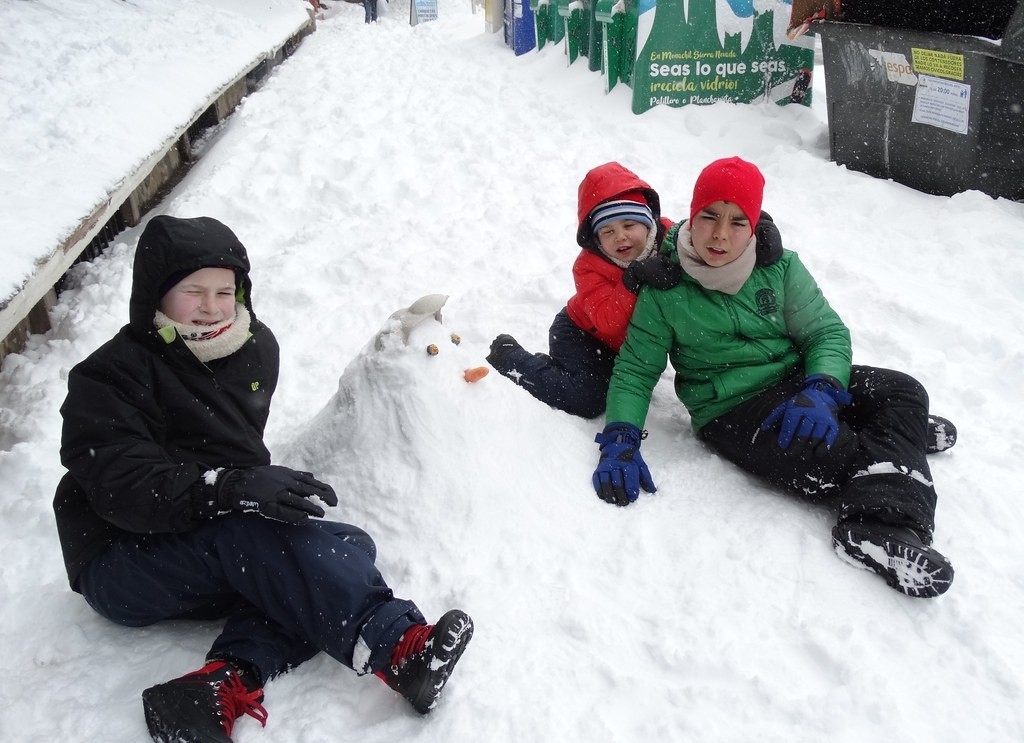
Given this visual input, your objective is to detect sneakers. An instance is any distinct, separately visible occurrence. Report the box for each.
[928,408,956,454]
[831,510,953,599]
[370,609,472,716]
[145,658,268,743]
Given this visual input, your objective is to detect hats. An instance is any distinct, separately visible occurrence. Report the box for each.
[688,155,764,238]
[591,187,652,235]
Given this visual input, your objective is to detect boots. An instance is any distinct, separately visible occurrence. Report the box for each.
[487,333,542,382]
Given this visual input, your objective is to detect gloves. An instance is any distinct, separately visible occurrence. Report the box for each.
[761,375,851,452]
[592,421,657,505]
[755,215,784,266]
[206,458,338,523]
[625,255,677,295]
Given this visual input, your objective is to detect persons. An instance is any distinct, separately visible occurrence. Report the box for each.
[487,157,958,599]
[52,216,473,743]
